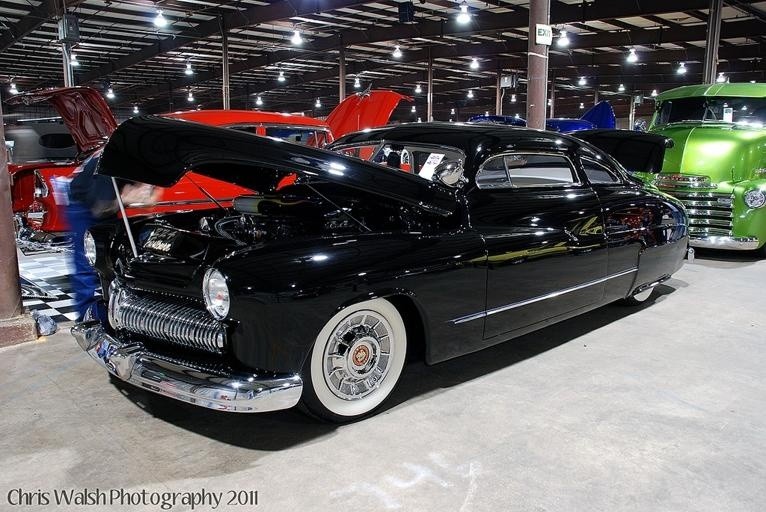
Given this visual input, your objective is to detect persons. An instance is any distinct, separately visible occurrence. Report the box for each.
[59,144,165,328]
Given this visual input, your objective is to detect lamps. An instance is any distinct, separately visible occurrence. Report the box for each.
[8,0,755,124]
